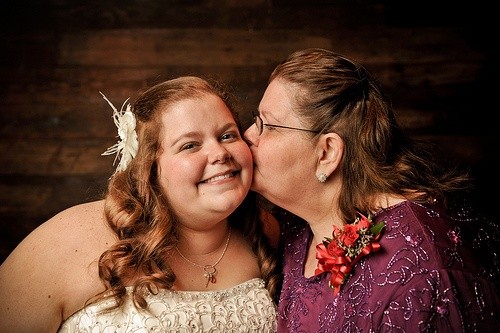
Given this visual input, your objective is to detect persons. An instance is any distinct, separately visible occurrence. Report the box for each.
[243,47,500,333]
[0,76,280,333]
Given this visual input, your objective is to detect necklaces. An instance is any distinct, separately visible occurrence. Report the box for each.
[172,225,232,286]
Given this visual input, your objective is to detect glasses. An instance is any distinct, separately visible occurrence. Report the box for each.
[251,110,327,136]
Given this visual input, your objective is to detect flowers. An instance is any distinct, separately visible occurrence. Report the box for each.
[99,90,138,179]
[314,212,384,295]
